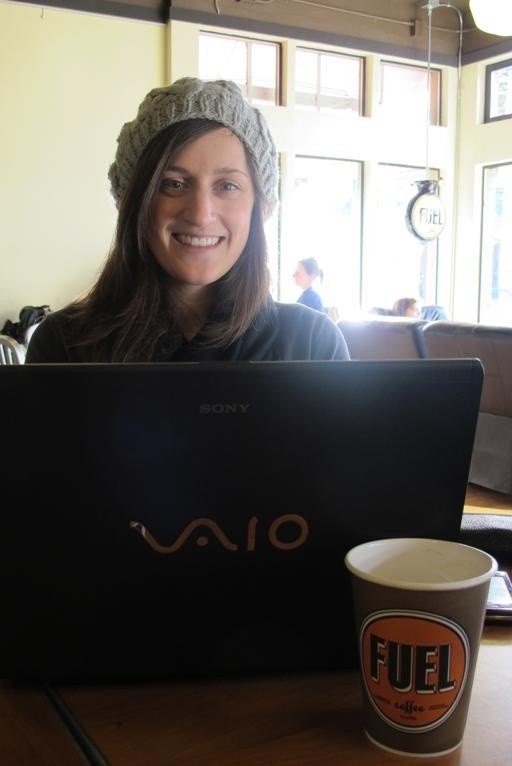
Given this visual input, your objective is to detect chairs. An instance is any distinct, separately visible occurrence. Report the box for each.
[337,314,511,496]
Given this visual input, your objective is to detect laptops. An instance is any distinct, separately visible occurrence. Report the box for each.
[0,357,486,691]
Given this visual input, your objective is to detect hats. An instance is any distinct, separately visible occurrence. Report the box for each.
[108,76,280,222]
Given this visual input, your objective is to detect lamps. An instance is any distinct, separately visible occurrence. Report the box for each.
[406,1,447,242]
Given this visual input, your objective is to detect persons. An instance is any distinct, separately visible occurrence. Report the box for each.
[393,297,422,318]
[25,75,349,359]
[293,257,324,311]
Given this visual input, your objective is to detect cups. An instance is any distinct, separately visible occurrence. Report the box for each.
[345,538,499,758]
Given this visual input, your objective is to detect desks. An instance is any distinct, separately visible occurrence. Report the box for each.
[0,620,512,766]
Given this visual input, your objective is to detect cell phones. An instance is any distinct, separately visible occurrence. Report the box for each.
[484,571,512,625]
